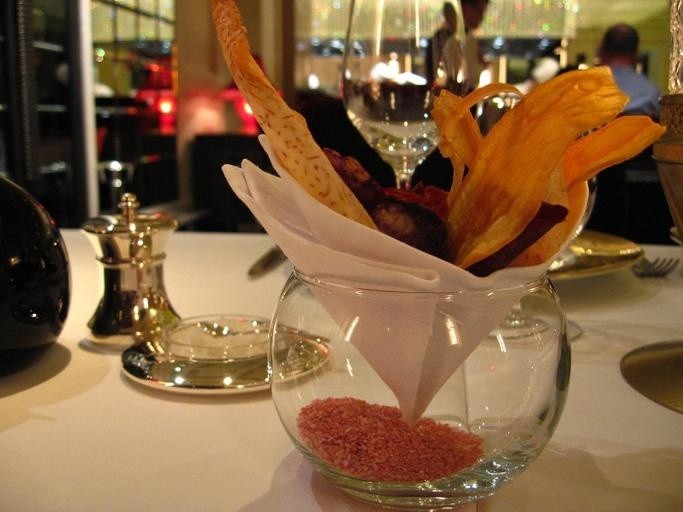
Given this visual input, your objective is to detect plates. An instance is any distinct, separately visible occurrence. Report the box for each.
[120,327,331,396]
[550,228,642,282]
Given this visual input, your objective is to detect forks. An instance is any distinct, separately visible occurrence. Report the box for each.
[631,253,681,280]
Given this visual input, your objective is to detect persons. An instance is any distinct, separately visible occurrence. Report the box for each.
[518,51,560,95]
[426,0,489,120]
[596,23,661,122]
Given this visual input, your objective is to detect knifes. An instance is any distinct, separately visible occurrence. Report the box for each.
[246,241,286,277]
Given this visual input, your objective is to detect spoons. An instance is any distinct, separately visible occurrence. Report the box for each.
[198,322,332,346]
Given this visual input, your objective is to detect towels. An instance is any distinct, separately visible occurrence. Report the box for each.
[220,133,560,430]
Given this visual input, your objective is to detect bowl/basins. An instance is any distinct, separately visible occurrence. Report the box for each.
[164,313,295,384]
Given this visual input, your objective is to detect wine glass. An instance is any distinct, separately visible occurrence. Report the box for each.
[469,95,553,338]
[338,0,465,190]
